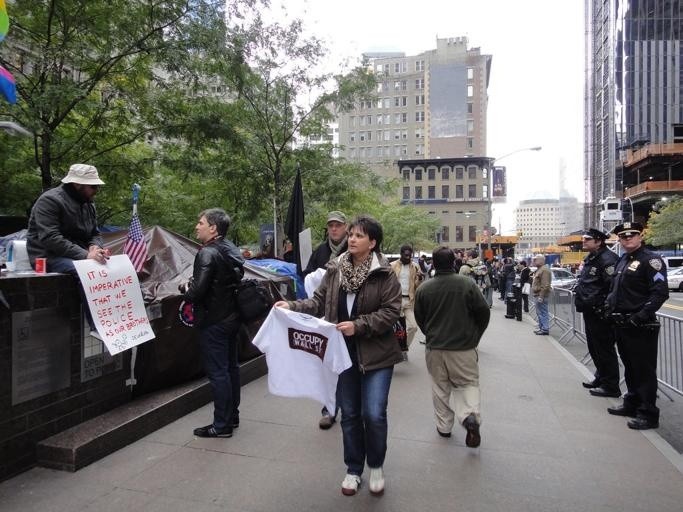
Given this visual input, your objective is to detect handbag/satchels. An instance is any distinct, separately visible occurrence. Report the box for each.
[224,278,274,324]
[521,282,531,295]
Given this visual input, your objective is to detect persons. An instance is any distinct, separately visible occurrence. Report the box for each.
[179,208,244,438]
[261,235,273,259]
[386,246,583,363]
[25,162,111,341]
[274,214,402,498]
[573,229,626,398]
[601,221,668,430]
[282,237,293,263]
[301,210,352,431]
[414,246,489,447]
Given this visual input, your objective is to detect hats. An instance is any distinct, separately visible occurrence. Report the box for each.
[59,162,106,187]
[531,253,545,259]
[325,210,347,226]
[581,228,610,240]
[613,221,644,238]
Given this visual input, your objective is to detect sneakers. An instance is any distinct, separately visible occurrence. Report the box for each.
[318,413,336,430]
[368,466,386,495]
[401,352,408,361]
[533,330,549,335]
[498,298,504,301]
[460,415,482,448]
[192,422,239,439]
[436,425,451,438]
[89,330,103,342]
[340,472,362,496]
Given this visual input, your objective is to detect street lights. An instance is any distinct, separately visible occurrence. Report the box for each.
[465,145,542,273]
[622,195,634,225]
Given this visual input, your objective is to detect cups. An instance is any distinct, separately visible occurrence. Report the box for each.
[6,262,16,273]
[35,258,47,274]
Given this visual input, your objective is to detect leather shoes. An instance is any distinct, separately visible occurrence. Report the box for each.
[607,403,637,418]
[626,414,660,430]
[589,387,621,398]
[582,381,599,388]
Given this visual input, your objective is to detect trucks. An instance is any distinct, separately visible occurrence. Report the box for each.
[382,252,437,279]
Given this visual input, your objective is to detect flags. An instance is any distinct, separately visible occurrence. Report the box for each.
[121,211,149,275]
[284,170,304,279]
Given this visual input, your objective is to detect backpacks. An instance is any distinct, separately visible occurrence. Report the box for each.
[463,260,489,296]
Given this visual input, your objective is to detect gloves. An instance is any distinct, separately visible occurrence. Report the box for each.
[625,313,643,330]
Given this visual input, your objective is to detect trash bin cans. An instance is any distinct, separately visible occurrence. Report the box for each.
[504,293,516,319]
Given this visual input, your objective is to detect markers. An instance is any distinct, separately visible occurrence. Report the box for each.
[100,253,110,260]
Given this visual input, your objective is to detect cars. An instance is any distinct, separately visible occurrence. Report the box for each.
[666,267,683,292]
[516,261,578,304]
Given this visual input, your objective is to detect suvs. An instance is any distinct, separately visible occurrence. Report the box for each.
[660,254,683,274]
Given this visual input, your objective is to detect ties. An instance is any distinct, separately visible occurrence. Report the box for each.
[608,254,631,313]
